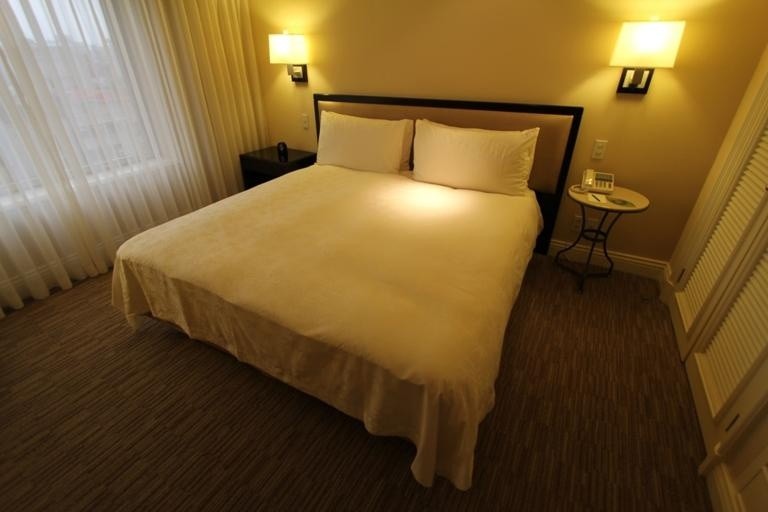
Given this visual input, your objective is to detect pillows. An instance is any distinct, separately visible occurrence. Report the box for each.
[315,108,411,176]
[409,117,539,197]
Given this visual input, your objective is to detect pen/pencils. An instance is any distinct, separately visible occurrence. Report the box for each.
[591,194,600,201]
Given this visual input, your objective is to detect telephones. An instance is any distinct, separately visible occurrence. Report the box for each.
[570,168,614,194]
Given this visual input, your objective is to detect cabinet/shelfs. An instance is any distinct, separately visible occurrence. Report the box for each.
[672,48,767,458]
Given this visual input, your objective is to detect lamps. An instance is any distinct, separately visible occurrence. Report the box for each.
[267,31,309,84]
[607,19,686,95]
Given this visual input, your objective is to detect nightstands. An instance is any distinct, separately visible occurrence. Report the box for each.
[238,145,317,190]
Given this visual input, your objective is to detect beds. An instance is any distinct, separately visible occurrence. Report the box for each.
[109,92,587,493]
